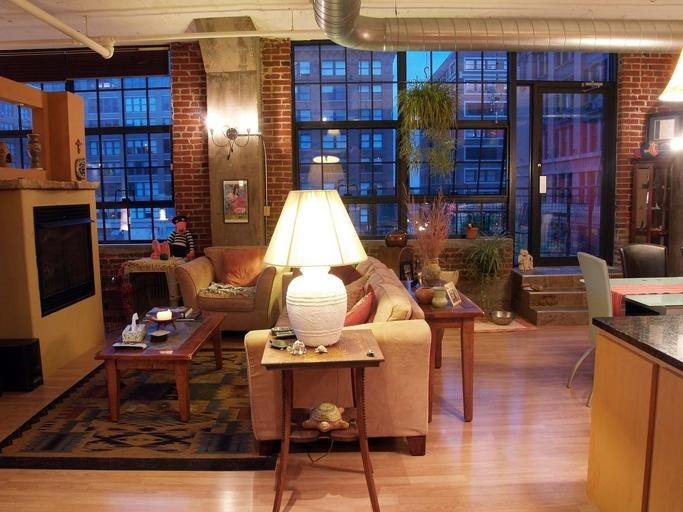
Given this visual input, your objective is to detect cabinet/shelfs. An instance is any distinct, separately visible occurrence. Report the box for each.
[629,158,670,247]
[0,76,104,380]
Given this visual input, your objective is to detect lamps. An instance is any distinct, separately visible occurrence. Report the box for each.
[264,190,367,348]
[658,45,683,151]
[308,155,345,188]
[204,104,260,160]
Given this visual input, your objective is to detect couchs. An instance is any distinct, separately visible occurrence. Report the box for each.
[243,256,430,456]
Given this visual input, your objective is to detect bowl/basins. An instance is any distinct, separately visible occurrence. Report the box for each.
[491,310,514,324]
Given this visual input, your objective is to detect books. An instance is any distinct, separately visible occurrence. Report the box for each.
[142,305,202,322]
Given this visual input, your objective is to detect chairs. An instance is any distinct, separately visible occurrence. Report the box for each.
[566,252,613,407]
[620,244,667,278]
[176,246,290,332]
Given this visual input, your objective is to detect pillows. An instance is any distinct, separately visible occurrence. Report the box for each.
[223,248,262,287]
[327,265,372,327]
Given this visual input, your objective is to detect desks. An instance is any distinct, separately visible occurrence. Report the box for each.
[409,281,485,424]
[260,330,385,512]
[123,255,186,320]
[578,276,683,316]
[585,314,683,512]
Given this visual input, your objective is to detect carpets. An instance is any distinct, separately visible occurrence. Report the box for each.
[0,348,277,470]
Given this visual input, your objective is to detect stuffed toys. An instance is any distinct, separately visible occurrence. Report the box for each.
[301,403,349,433]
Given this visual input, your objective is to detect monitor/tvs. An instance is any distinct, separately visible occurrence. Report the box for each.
[33,204,97,318]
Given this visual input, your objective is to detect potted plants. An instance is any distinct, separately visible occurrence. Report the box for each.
[394,80,510,322]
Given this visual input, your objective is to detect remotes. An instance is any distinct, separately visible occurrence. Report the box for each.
[271,326,294,333]
[275,331,296,339]
[111,342,147,349]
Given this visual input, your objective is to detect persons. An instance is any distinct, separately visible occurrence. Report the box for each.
[151,216,194,261]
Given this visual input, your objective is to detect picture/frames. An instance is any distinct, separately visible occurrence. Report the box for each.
[223,178,249,224]
